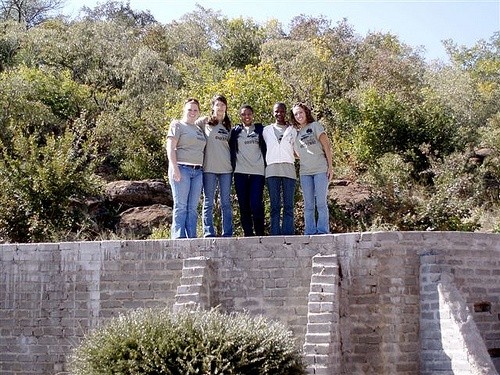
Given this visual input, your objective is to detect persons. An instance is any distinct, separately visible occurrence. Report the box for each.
[194,94,298,237]
[166,99,206,239]
[291,103,333,235]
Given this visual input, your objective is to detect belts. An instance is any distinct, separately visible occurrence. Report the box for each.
[178,164,204,169]
